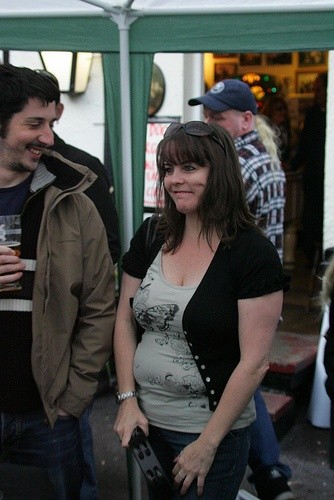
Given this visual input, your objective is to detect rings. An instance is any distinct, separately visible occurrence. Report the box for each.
[178,471,184,479]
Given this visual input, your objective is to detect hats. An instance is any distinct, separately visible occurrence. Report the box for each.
[189,79,257,116]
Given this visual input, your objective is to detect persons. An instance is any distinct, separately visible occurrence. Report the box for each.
[0,62,123,500]
[187,78,295,500]
[114,120,286,500]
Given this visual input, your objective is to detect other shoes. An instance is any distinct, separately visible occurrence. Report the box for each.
[254,469,294,500]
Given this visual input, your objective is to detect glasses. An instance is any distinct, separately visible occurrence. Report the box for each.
[163,119,225,148]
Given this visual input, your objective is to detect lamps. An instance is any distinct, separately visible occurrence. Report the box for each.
[39,50,93,94]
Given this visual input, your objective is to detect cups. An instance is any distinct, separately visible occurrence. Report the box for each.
[0,214,22,290]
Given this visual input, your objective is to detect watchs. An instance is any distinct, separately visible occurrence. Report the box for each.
[115,391,138,405]
